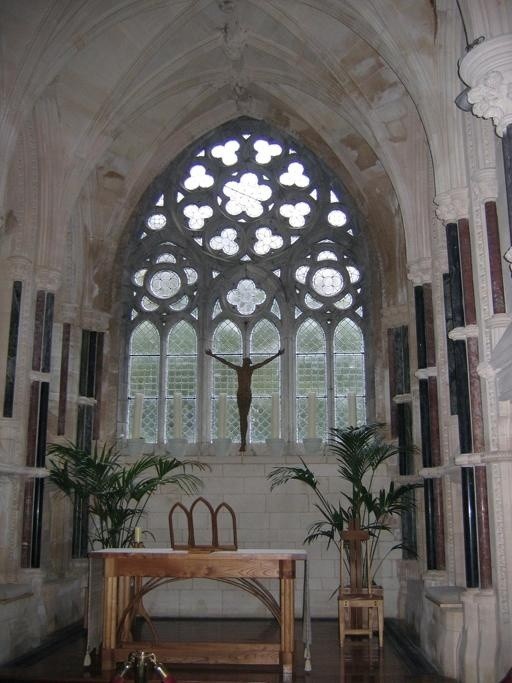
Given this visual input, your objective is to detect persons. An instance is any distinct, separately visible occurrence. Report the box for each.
[205,347,284,451]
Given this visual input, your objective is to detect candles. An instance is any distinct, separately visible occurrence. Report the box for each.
[135,527,141,544]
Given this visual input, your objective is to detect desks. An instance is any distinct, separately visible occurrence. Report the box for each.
[86,547,308,673]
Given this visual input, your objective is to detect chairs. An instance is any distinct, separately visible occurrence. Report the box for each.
[336,518,384,648]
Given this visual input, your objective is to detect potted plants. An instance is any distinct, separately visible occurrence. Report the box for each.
[264,417,428,638]
[39,436,210,638]
